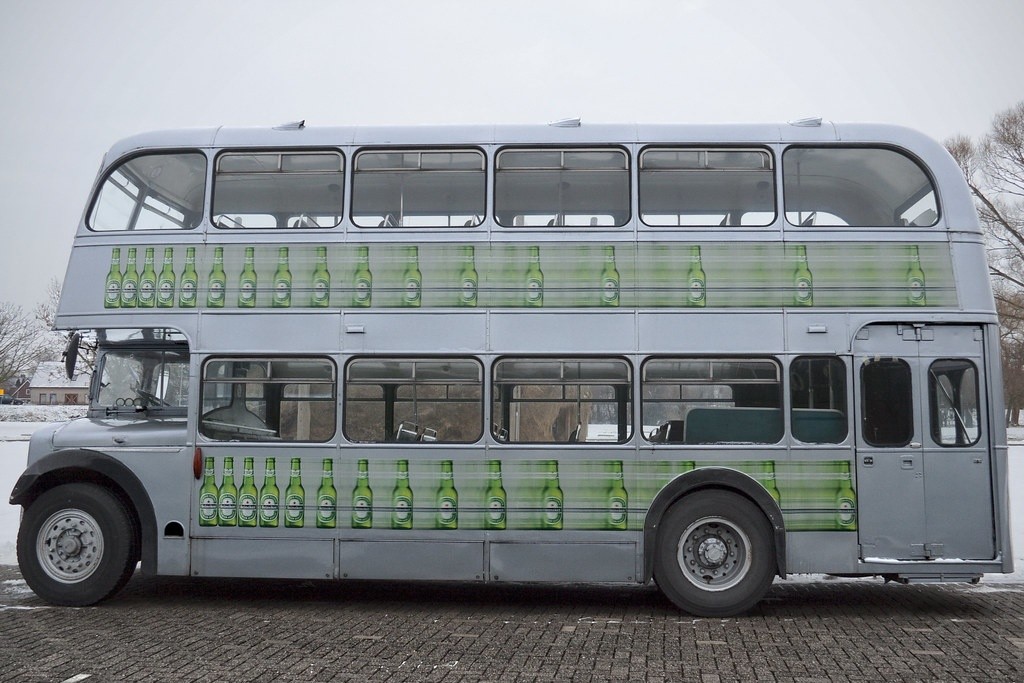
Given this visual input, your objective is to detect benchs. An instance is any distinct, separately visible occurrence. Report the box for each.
[685,409,846,446]
[218,209,909,228]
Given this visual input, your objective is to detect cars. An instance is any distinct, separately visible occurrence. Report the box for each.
[0,394,23,405]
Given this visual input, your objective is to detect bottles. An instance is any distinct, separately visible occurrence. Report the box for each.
[459,246,479,307]
[352,460,372,529]
[606,460,628,531]
[685,461,695,473]
[352,246,372,308]
[104,248,122,308]
[218,457,238,527]
[238,457,258,526]
[157,248,176,307]
[179,248,199,308]
[485,460,507,530]
[907,245,925,306]
[199,457,217,527]
[238,247,257,307]
[600,246,619,307]
[259,457,280,526]
[311,247,329,307]
[541,461,564,530]
[834,461,856,531]
[761,461,778,508]
[272,247,292,307]
[121,248,138,307]
[207,247,226,307]
[402,246,422,307]
[524,246,544,307]
[138,248,157,308]
[793,245,813,307]
[391,459,413,529]
[284,458,304,527]
[687,244,705,307]
[316,458,336,529]
[435,460,458,529]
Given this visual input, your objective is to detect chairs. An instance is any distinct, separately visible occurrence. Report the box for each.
[393,421,437,443]
[569,422,582,442]
[493,423,508,442]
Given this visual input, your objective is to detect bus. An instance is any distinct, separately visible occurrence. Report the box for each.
[9,117,1014,617]
